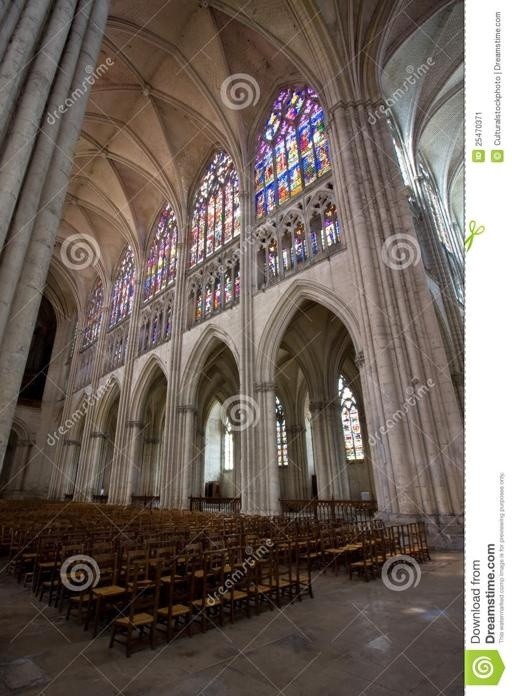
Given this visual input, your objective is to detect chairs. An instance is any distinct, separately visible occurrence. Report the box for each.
[1,499,431,656]
